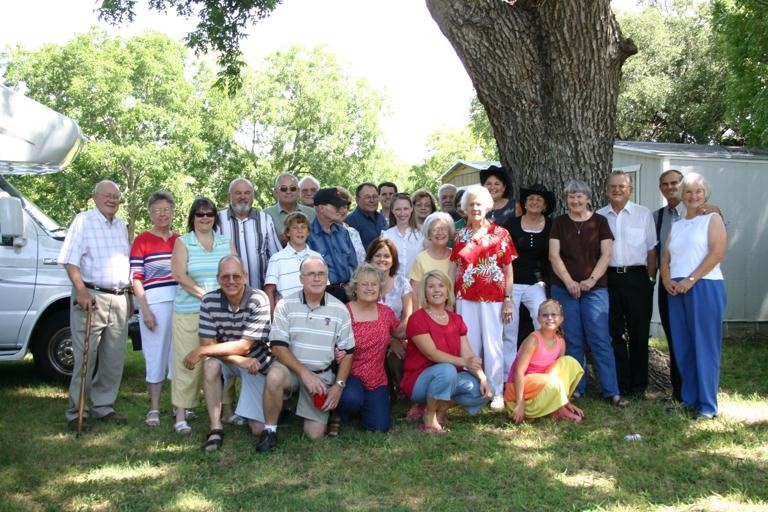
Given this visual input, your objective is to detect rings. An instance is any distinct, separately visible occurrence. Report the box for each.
[703,209,706,212]
[508,316,511,318]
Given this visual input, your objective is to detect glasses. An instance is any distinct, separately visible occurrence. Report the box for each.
[609,185,633,191]
[526,197,544,202]
[433,228,450,233]
[95,192,120,199]
[393,192,410,196]
[194,212,215,217]
[539,312,562,318]
[217,271,244,282]
[150,208,172,213]
[301,271,327,277]
[357,195,378,200]
[276,185,296,192]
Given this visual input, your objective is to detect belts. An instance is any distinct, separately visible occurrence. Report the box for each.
[85,283,129,295]
[312,364,331,374]
[328,282,345,288]
[607,265,646,274]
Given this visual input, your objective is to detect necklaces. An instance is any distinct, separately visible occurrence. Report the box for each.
[427,308,448,320]
[568,213,591,235]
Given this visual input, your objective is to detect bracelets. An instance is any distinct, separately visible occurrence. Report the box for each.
[590,274,597,282]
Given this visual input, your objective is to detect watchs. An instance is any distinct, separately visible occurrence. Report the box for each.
[688,275,696,284]
[649,275,656,283]
[504,296,512,301]
[335,380,346,387]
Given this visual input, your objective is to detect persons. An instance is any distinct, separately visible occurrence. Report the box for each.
[549,178,630,408]
[262,212,329,323]
[334,185,367,267]
[305,187,360,305]
[411,189,437,232]
[659,173,728,421]
[171,197,249,436]
[365,238,413,401]
[409,211,459,313]
[380,193,426,278]
[448,184,519,413]
[503,298,585,421]
[594,170,657,399]
[57,180,131,434]
[344,183,388,251]
[438,183,461,221]
[503,183,557,388]
[480,165,524,226]
[377,181,397,227]
[453,189,468,230]
[216,178,284,290]
[182,254,274,453]
[651,170,724,400]
[255,257,355,453]
[328,266,408,438]
[400,270,492,434]
[297,175,321,208]
[130,191,196,426]
[264,173,317,247]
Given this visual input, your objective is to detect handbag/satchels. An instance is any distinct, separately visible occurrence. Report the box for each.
[656,208,664,267]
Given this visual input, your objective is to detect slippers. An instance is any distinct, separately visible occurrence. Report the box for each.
[551,409,581,422]
[437,415,449,424]
[419,424,442,434]
[406,408,421,422]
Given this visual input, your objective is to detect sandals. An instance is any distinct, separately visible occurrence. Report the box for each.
[611,395,629,407]
[145,410,160,427]
[569,391,582,401]
[204,429,223,451]
[173,406,196,421]
[174,422,191,435]
[329,417,340,436]
[222,414,247,425]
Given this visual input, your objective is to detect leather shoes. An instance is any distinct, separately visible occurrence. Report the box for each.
[661,395,673,403]
[666,402,687,413]
[69,418,88,431]
[100,412,127,424]
[691,411,714,421]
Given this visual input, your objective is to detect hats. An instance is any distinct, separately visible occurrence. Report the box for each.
[480,165,509,197]
[520,184,556,216]
[314,188,351,207]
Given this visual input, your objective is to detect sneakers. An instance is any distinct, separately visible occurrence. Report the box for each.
[256,429,278,452]
[490,396,505,412]
